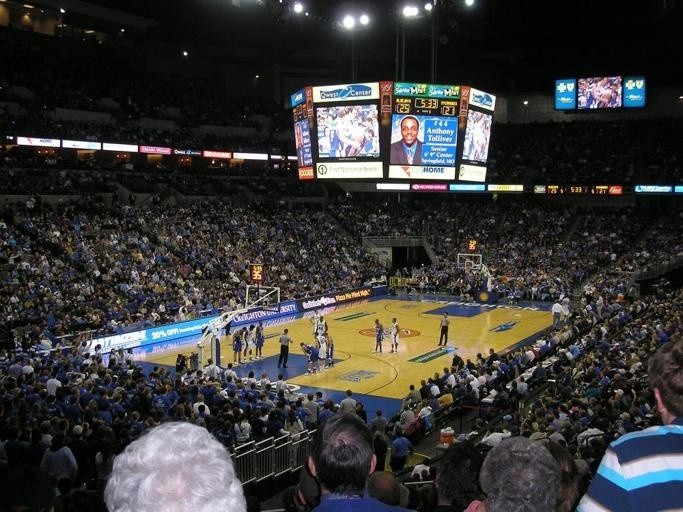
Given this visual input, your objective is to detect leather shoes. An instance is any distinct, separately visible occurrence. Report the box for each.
[283,365,288,368]
[277,364,280,368]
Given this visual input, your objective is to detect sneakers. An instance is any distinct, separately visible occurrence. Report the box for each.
[234,355,263,365]
[304,370,313,376]
[312,368,317,373]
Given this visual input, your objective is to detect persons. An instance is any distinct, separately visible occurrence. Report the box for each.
[1,36,682,512]
